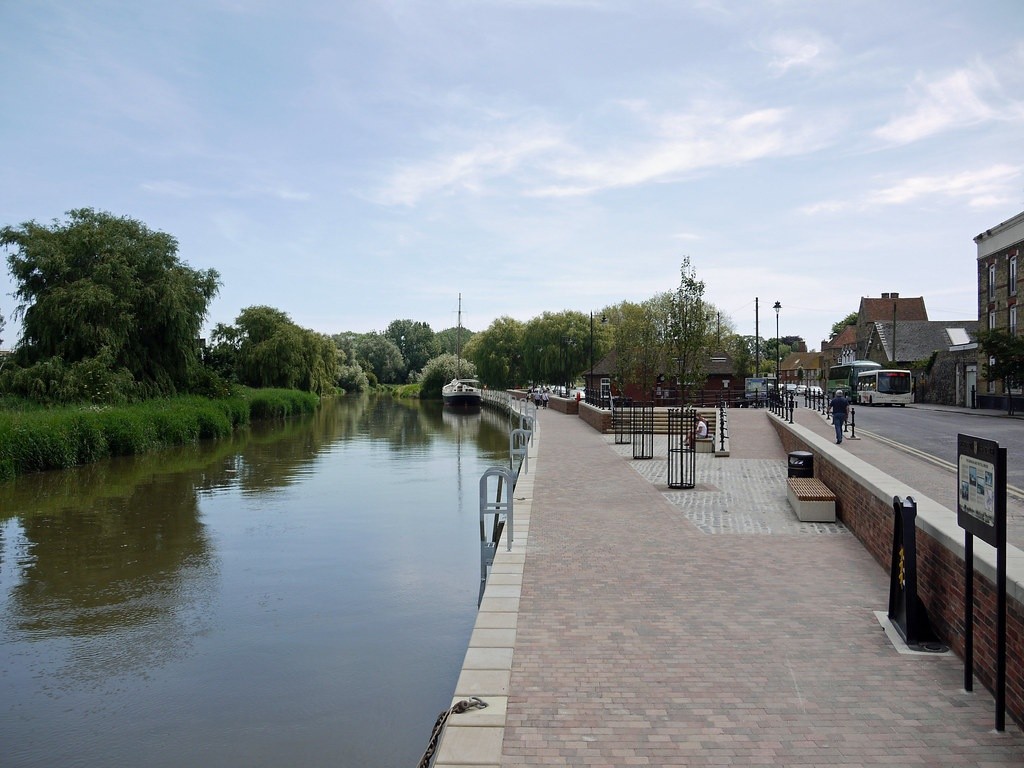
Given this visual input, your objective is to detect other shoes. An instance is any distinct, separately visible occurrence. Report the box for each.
[836,440,842,444]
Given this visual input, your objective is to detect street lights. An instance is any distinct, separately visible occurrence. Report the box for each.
[558,333,573,398]
[772,300,783,394]
[590,310,608,404]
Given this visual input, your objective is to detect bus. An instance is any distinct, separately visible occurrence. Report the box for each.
[855,370,913,407]
[826,360,881,404]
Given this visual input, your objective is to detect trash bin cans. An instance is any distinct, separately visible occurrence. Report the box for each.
[788,451,814,479]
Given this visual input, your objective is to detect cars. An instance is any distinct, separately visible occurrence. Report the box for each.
[767,383,824,399]
[533,384,567,398]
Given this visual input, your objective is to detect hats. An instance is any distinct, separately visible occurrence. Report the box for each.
[836,389,843,396]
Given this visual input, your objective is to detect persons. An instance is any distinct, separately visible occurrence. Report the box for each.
[526,387,553,410]
[684,418,707,446]
[684,413,710,438]
[827,389,850,444]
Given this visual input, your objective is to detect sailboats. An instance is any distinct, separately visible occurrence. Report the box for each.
[442,292,483,408]
